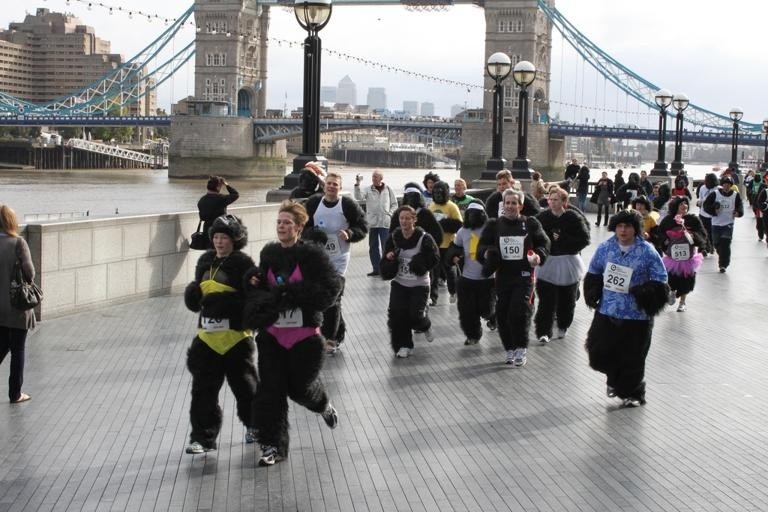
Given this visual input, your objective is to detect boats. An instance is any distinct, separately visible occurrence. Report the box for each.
[740,159,763,169]
[711,167,721,172]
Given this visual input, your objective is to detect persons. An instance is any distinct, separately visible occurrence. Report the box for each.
[0,202,37,402]
[477,168,591,367]
[303,172,368,355]
[565,161,590,206]
[198,173,239,250]
[381,172,499,358]
[355,170,399,276]
[185,215,257,455]
[583,207,669,406]
[694,162,768,271]
[240,199,338,468]
[289,160,327,199]
[594,169,708,312]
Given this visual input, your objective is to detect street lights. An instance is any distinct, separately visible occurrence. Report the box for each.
[762,118,768,167]
[669,90,689,177]
[276,0,333,190]
[649,86,674,176]
[509,57,538,179]
[725,104,744,173]
[480,50,512,181]
[463,87,472,119]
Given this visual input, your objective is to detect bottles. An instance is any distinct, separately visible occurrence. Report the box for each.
[317,220,327,233]
[274,275,286,285]
[527,249,536,268]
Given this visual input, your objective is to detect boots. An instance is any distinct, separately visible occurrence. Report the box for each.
[595,215,608,227]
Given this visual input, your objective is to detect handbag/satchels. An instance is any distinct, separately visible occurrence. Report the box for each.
[190,232,211,250]
[589,190,599,203]
[533,192,544,200]
[569,177,580,190]
[10,283,44,310]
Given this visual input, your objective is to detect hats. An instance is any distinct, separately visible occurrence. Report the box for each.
[209,213,247,250]
[608,208,644,236]
[720,176,734,186]
[631,194,653,212]
[669,196,690,214]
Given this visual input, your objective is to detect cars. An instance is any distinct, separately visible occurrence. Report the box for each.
[319,113,455,123]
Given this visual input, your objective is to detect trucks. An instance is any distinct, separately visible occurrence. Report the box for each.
[615,121,642,133]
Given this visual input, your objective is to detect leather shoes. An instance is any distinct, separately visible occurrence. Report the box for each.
[367,271,380,276]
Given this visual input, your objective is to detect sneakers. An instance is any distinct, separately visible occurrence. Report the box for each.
[429,278,456,306]
[186,440,217,454]
[464,327,483,345]
[607,381,645,407]
[505,347,526,366]
[668,291,687,312]
[558,328,567,339]
[245,428,259,443]
[487,315,496,330]
[720,266,726,272]
[320,399,338,429]
[258,444,288,466]
[538,335,551,343]
[324,339,340,357]
[397,346,415,358]
[425,323,433,342]
[10,392,32,403]
